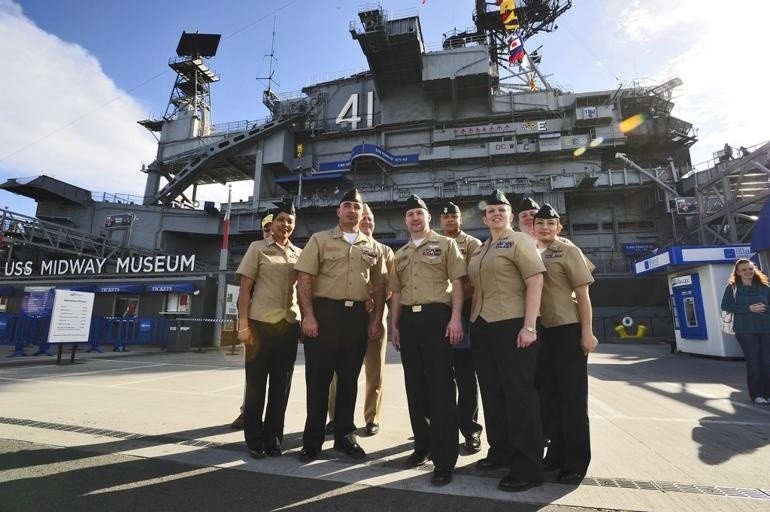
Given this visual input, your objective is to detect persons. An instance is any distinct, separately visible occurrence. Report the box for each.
[232,212,277,432]
[467,188,548,493]
[390,194,468,486]
[233,199,302,459]
[515,196,596,278]
[320,202,395,437]
[721,257,770,405]
[436,200,482,456]
[292,188,388,466]
[530,202,600,486]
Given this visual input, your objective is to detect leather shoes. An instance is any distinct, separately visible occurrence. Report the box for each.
[249,446,265,458]
[326,421,334,434]
[265,440,281,456]
[412,454,428,467]
[544,456,564,471]
[478,458,493,468]
[466,435,481,453]
[232,414,245,430]
[432,466,453,486]
[334,444,366,458]
[557,471,581,484]
[365,423,379,436]
[300,445,322,462]
[498,477,544,491]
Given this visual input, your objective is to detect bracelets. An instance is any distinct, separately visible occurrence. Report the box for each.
[237,328,249,332]
[524,325,538,334]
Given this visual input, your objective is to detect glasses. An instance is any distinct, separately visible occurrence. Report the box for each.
[263,228,271,233]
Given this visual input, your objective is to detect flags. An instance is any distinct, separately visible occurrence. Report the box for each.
[499,0,519,30]
[504,31,524,63]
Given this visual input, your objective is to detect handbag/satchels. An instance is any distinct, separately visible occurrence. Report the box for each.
[720,283,737,335]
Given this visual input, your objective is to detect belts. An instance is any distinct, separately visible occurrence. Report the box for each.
[402,304,446,313]
[333,300,364,307]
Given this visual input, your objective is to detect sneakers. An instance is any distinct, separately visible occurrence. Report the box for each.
[753,398,767,403]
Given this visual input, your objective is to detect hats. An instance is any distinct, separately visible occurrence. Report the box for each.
[403,194,427,213]
[516,197,540,214]
[440,202,460,214]
[340,187,362,203]
[363,203,372,214]
[262,214,273,226]
[280,198,295,215]
[488,189,510,205]
[535,203,560,219]
